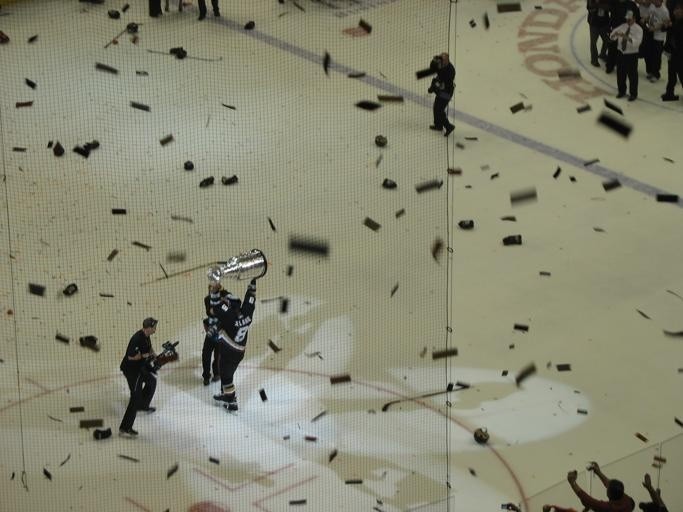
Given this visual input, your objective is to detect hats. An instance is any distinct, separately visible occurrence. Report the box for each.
[142,318,158,328]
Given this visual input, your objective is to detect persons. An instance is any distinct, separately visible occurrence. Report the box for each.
[586,0,683,100]
[502,460,669,512]
[209,277,262,415]
[201,282,232,385]
[118,317,159,437]
[427,52,456,136]
[197,0,222,20]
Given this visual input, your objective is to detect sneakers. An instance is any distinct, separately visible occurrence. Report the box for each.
[119,428,138,435]
[139,409,155,412]
[213,394,236,402]
[223,404,237,411]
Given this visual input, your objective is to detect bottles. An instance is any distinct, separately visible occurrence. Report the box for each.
[458,220,473,228]
[198,177,213,186]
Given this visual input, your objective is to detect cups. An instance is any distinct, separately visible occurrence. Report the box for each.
[221,175,237,184]
[78,335,96,344]
[92,428,111,439]
[502,235,521,245]
[107,10,118,18]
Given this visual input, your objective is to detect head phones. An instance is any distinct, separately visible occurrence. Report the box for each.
[151,319,156,327]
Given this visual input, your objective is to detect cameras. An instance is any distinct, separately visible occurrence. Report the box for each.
[501,504,512,510]
[617,30,623,35]
[586,462,594,470]
